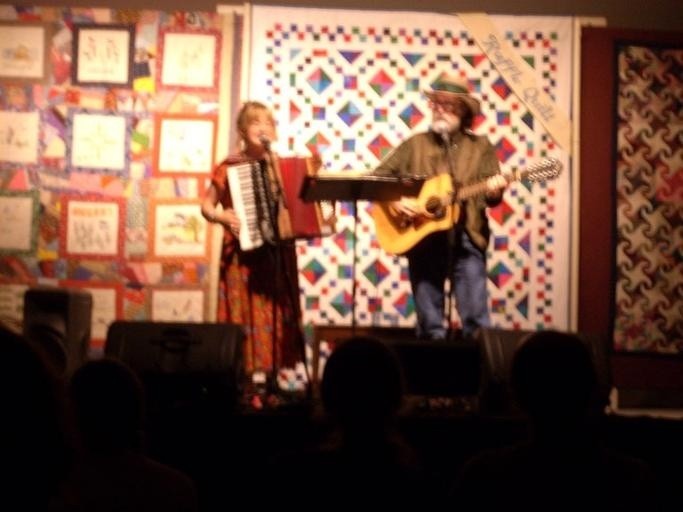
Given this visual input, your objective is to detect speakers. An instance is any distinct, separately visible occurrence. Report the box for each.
[23,285,92,377]
[104,319,245,391]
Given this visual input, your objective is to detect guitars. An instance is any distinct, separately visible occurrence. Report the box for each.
[370,157,562,256]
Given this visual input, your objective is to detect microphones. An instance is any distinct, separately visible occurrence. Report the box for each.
[260,134,277,156]
[437,120,454,157]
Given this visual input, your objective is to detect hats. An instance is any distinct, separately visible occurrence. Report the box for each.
[422,73,480,117]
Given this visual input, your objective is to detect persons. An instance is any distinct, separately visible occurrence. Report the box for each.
[0,323,78,512]
[459,329,667,512]
[201,101,337,409]
[46,358,202,512]
[254,335,468,512]
[369,73,510,339]
